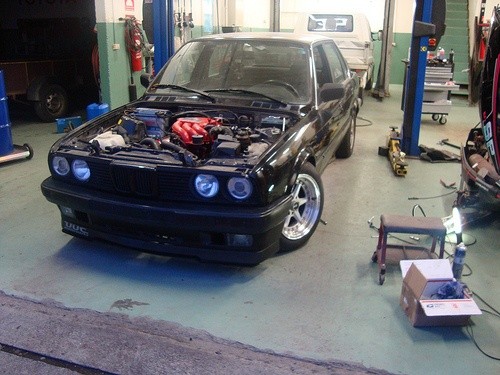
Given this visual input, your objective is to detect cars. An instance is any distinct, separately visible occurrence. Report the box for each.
[41,31,361,267]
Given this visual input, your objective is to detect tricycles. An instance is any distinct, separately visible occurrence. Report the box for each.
[0,44,96,121]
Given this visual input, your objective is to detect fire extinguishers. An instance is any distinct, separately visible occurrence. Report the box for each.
[129,19,142,71]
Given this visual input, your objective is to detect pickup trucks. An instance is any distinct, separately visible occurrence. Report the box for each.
[295,10,382,92]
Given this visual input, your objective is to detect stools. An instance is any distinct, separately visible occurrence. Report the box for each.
[373,214,446,285]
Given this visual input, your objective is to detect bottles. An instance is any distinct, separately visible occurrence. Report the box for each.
[452,242,466,280]
[437,46,443,60]
[449,48,454,64]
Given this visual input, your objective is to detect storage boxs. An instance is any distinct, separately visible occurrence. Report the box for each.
[399,258,482,328]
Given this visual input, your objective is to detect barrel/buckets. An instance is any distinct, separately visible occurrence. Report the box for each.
[87,102,109,121]
[0,68,14,157]
